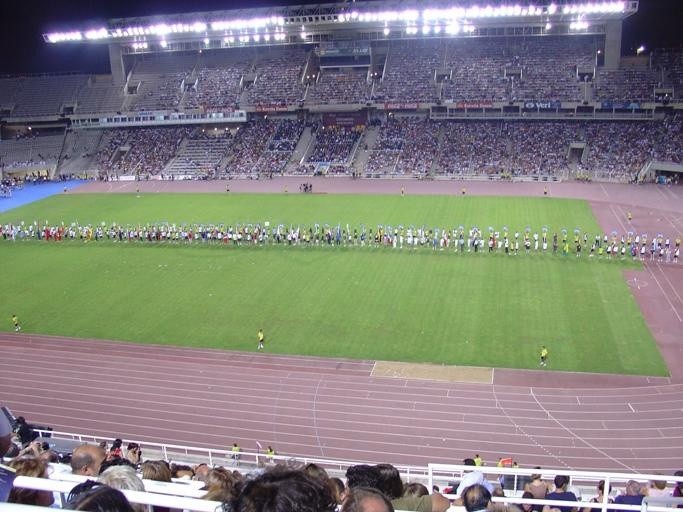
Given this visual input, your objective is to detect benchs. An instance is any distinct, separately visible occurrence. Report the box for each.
[0,50,259,181]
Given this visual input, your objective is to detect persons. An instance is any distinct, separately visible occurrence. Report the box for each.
[538,346,547,368]
[11,315,21,332]
[1,40,682,199]
[626,210,632,223]
[257,329,264,351]
[1,216,681,266]
[0,404,683,512]
